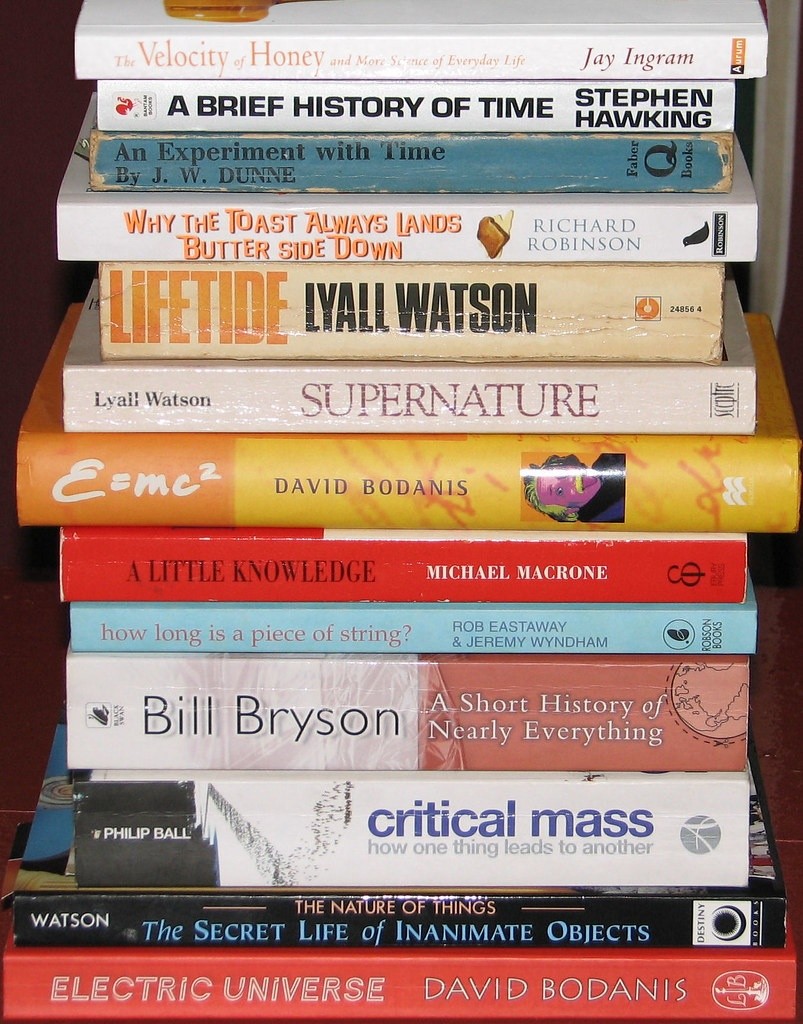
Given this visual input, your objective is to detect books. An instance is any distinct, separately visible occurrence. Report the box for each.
[1,817,799,1019]
[15,309,799,532]
[55,123,764,263]
[63,657,755,767]
[71,771,747,896]
[84,136,737,192]
[67,598,762,657]
[51,523,751,609]
[61,273,757,438]
[74,0,772,77]
[94,81,741,131]
[96,263,724,365]
[13,723,782,949]
